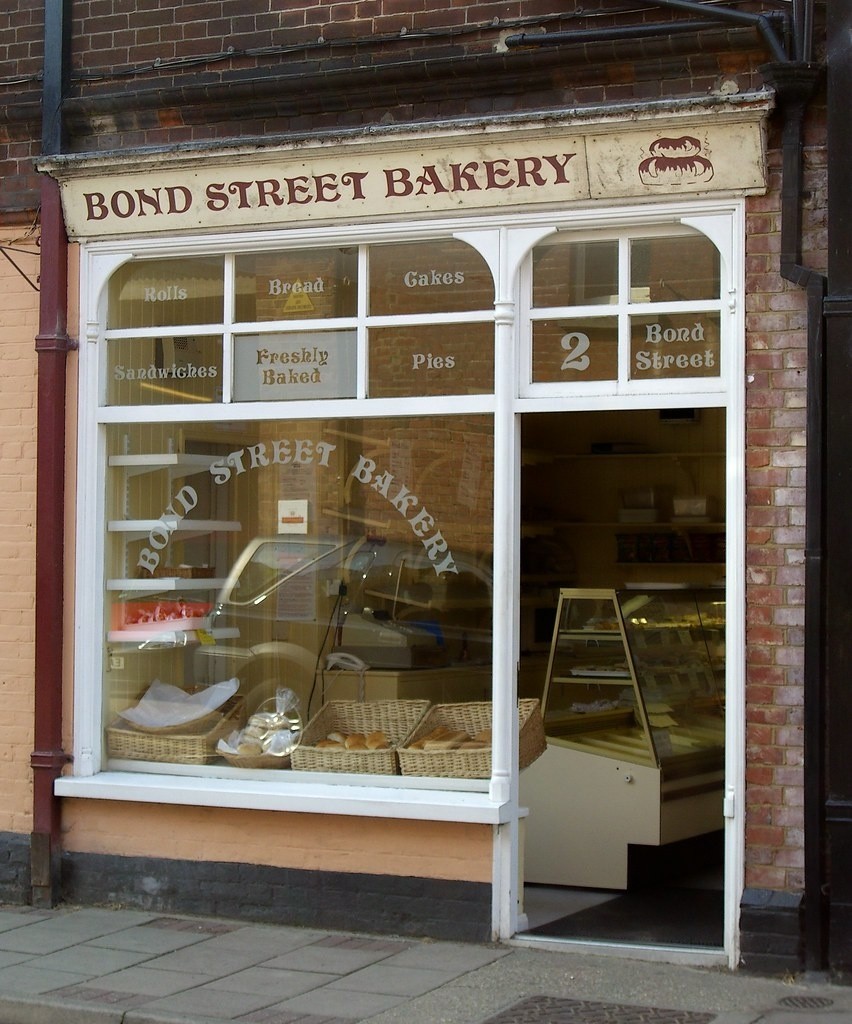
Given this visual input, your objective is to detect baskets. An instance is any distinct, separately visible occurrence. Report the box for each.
[289,699,432,774]
[216,697,303,767]
[104,681,245,766]
[395,697,546,779]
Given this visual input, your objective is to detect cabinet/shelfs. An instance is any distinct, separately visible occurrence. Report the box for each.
[107,433,242,644]
[319,664,492,702]
[539,586,727,769]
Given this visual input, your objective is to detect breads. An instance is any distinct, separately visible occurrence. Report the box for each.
[409,724,492,753]
[237,714,288,758]
[314,727,387,754]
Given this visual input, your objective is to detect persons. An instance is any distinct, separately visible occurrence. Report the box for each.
[400,582,453,671]
[443,569,490,665]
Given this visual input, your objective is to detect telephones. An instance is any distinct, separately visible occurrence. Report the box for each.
[325,651,370,672]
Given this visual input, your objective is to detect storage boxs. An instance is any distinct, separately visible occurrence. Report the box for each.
[616,484,663,510]
[672,493,718,517]
[616,508,661,525]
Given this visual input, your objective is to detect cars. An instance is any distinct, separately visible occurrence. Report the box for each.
[190,535,493,757]
